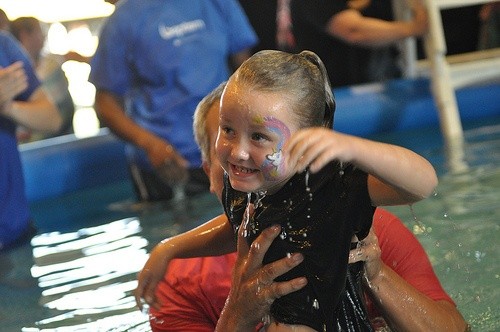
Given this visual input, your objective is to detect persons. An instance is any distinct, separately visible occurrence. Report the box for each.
[0,6,95,259]
[291,0,499,86]
[89,0,264,203]
[136,50,438,332]
[147,79,468,332]
